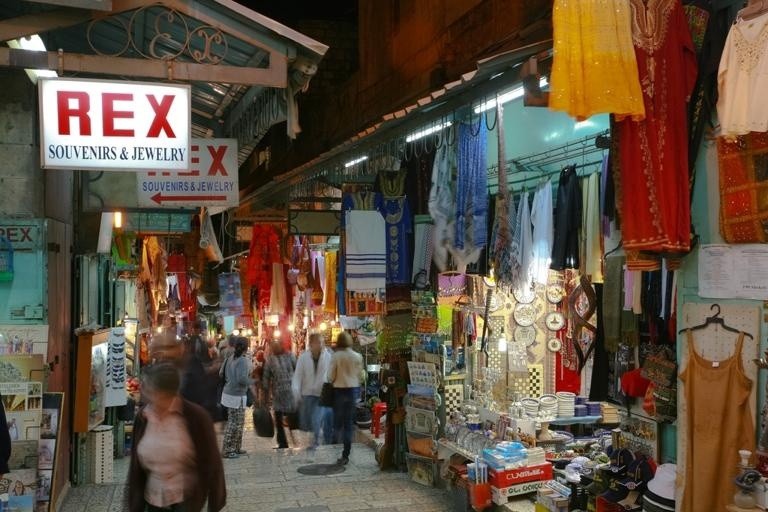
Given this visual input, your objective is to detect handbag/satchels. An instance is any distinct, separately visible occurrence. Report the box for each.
[640,353,675,388]
[643,381,677,425]
[319,383,334,407]
[253,409,274,437]
[216,383,224,403]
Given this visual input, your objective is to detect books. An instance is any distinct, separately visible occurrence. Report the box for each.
[402,348,443,487]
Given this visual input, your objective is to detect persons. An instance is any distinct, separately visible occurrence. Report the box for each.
[149,322,369,466]
[122,363,227,512]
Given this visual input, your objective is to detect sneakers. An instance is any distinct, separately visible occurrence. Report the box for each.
[231,450,246,457]
[337,457,349,466]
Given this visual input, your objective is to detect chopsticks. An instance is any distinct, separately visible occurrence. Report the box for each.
[456,424,497,454]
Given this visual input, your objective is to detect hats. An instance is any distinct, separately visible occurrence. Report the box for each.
[642,463,678,511]
[583,448,652,511]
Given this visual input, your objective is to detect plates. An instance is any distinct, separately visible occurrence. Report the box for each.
[547,338,562,352]
[513,279,536,303]
[547,283,563,304]
[482,292,500,311]
[521,391,601,417]
[545,311,565,331]
[484,268,499,286]
[514,303,536,326]
[514,325,536,348]
